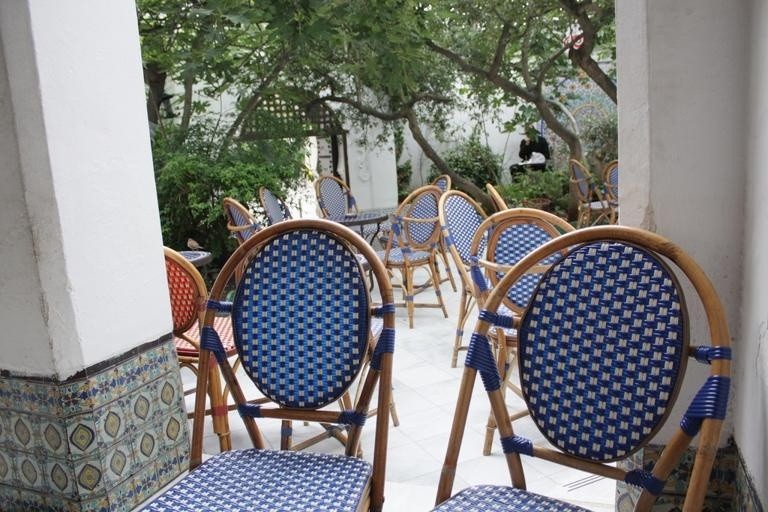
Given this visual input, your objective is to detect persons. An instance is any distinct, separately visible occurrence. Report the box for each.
[508,123,550,184]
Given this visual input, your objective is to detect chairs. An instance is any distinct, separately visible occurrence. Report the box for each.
[162,246,399,458]
[438,183,578,456]
[140,219,395,512]
[429,225,731,512]
[224,174,458,329]
[569,158,619,226]
